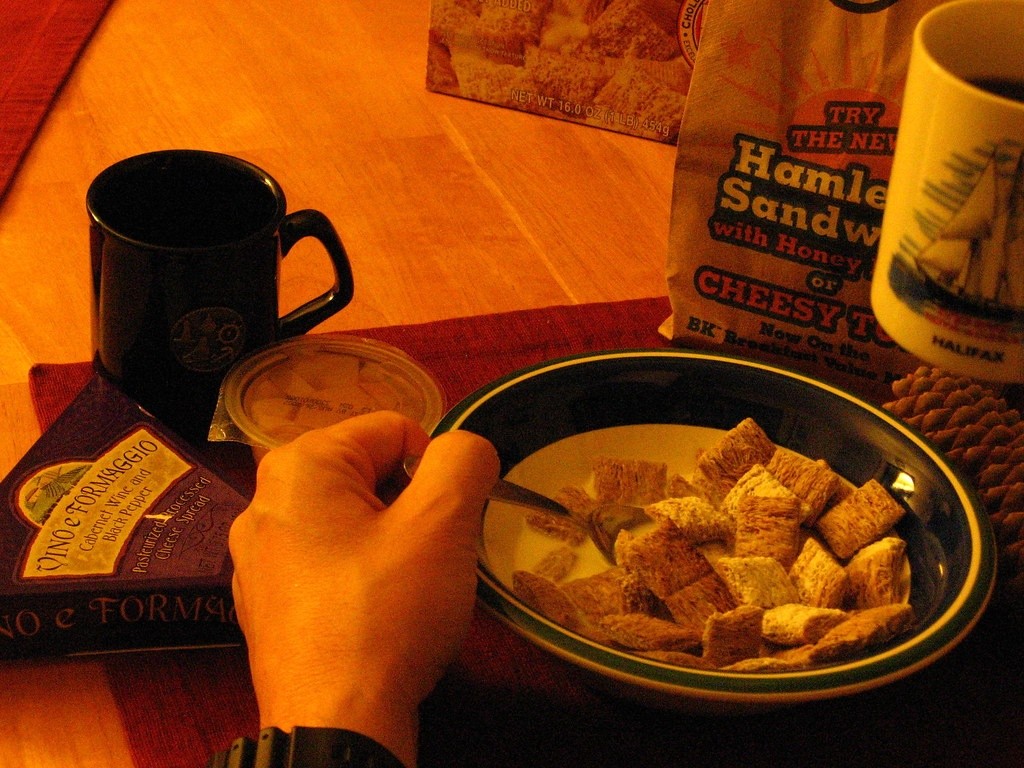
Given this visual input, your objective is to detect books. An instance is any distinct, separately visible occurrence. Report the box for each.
[1,372,265,658]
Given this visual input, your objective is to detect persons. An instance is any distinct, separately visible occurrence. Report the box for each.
[204,409,501,768]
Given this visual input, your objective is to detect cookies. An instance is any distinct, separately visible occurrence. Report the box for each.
[509,418,917,669]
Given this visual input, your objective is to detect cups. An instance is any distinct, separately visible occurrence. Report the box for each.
[84,150,353,416]
[869,0,1024,388]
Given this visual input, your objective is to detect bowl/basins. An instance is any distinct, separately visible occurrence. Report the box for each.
[426,348,998,704]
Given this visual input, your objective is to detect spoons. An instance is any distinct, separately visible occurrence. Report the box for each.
[400,453,673,565]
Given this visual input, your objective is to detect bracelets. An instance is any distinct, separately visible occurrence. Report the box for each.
[203,725,406,768]
